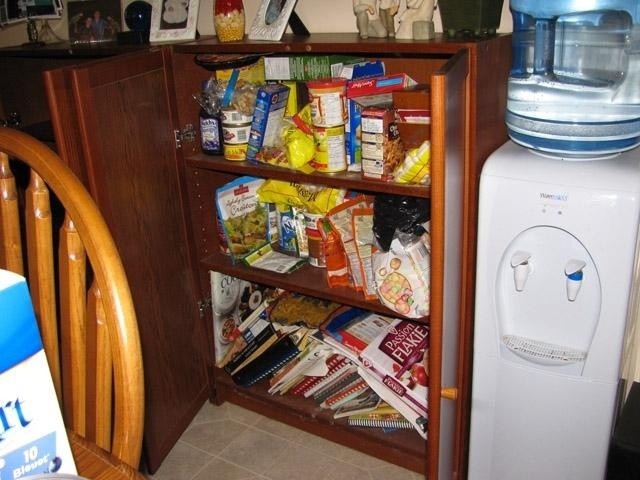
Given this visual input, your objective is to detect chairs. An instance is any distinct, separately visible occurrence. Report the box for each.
[0,128,147,477]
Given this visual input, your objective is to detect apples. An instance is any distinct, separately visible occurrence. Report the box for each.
[398,371,415,388]
[411,363,430,386]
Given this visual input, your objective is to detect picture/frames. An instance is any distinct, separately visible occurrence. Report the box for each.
[246,0,312,46]
[148,0,199,42]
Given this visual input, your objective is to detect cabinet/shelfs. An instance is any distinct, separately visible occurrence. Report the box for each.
[43,32,516,479]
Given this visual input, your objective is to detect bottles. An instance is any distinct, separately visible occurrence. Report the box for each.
[200,80,222,156]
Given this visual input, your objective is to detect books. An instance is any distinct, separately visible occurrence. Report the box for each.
[210,270,429,440]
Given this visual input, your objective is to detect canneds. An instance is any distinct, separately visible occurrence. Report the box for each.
[220,107,253,161]
[314,124,347,174]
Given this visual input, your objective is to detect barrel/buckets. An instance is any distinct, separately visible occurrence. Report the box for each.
[504,0,640,161]
